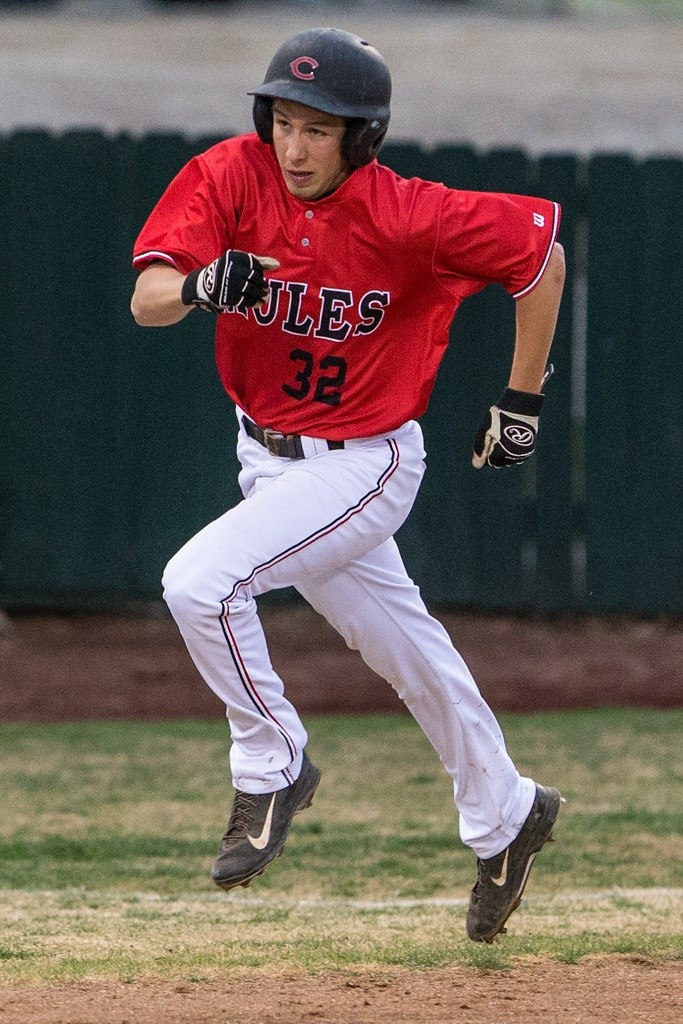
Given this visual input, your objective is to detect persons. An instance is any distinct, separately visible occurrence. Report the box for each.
[131,24,566,942]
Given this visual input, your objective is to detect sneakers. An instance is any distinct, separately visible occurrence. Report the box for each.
[466,783,561,943]
[213,749,320,891]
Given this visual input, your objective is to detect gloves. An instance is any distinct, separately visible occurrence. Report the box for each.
[181,248,280,314]
[472,387,546,468]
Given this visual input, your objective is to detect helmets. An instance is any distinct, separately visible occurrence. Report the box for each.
[246,27,392,167]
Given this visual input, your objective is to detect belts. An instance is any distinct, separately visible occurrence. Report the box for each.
[241,414,344,460]
